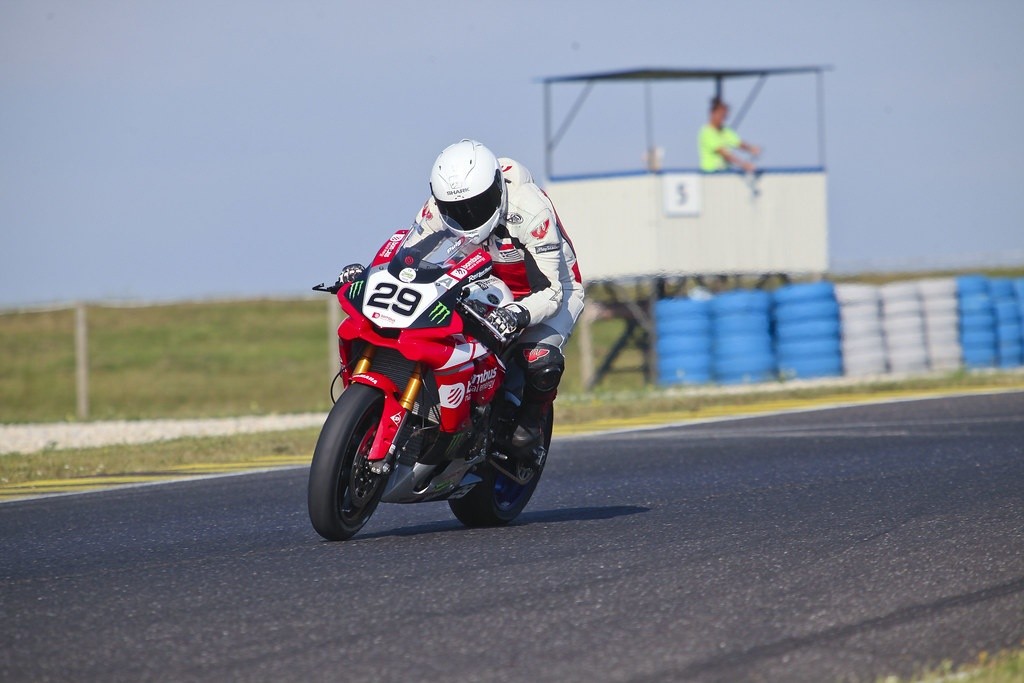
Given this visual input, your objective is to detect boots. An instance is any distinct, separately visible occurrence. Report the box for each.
[508,390,556,447]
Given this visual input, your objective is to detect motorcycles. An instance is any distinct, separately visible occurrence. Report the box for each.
[306,214,564,542]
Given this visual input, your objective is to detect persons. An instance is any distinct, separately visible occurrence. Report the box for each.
[697,97,761,175]
[338,138,584,451]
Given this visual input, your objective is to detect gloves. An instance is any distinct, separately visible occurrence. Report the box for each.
[486,303,529,340]
[338,264,365,284]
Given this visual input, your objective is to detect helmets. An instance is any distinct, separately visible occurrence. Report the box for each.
[430,139,505,246]
